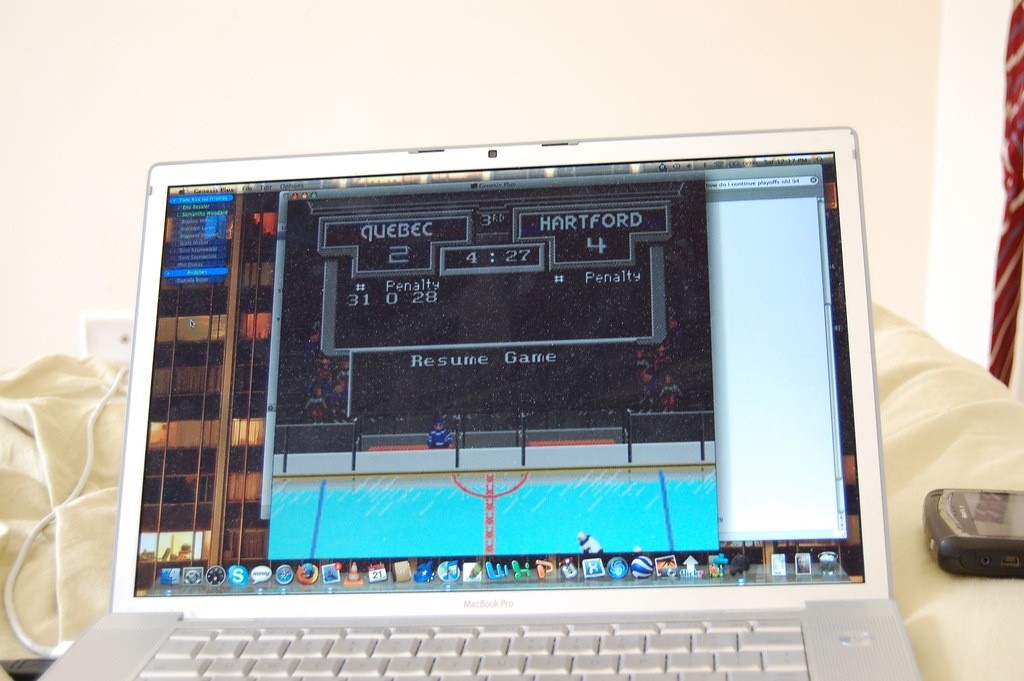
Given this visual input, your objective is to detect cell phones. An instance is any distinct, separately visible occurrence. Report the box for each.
[922,488,1024,580]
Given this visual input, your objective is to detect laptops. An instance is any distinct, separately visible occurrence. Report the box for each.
[31,127,919,681]
